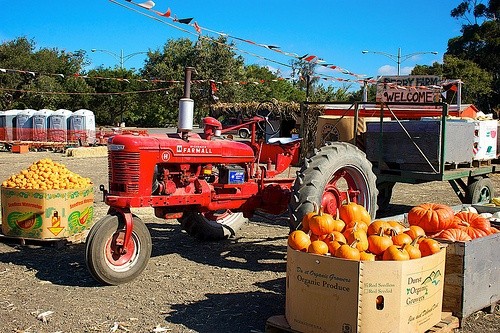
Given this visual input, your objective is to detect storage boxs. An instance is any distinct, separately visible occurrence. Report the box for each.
[365,118,498,166]
[316,115,391,149]
[285,242,446,333]
[0,184,95,240]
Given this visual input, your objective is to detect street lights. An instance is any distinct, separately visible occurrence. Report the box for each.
[362,48,438,76]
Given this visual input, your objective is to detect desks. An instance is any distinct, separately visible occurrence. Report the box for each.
[372,204,500,321]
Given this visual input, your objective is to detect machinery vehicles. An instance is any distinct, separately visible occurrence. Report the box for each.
[84,65,500,286]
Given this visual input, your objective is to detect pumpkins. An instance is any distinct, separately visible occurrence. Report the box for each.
[287,191,440,261]
[405,201,500,242]
[2,157,91,191]
